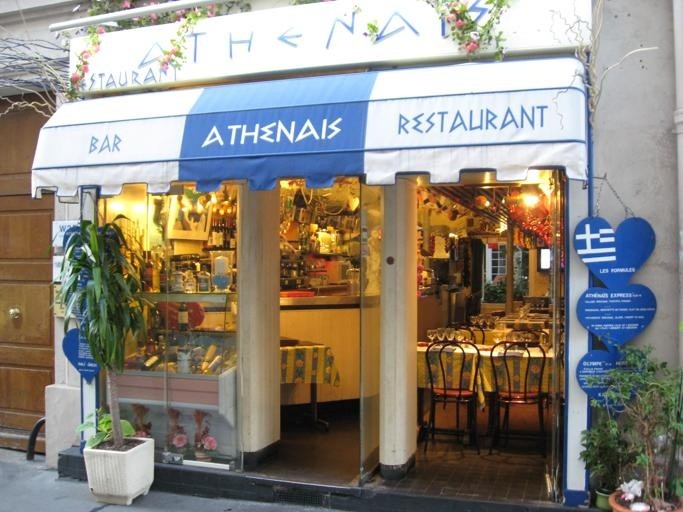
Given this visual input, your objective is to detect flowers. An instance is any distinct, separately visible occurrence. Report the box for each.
[132,402,218,450]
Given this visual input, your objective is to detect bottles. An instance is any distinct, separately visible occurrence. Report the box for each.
[124,335,235,375]
[281,255,307,290]
[173,265,210,292]
[178,303,189,331]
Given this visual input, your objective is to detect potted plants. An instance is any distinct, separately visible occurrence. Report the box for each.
[44,212,156,506]
[578,351,682,512]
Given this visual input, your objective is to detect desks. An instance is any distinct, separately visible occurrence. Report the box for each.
[271,337,334,434]
[417,340,555,454]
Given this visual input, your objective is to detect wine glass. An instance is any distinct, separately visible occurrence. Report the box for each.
[426,327,474,348]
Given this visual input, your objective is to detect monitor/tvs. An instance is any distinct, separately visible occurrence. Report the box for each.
[537,247,551,272]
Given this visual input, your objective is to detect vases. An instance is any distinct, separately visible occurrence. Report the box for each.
[193,444,213,461]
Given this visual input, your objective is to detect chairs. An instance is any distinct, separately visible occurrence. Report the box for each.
[423,317,548,457]
